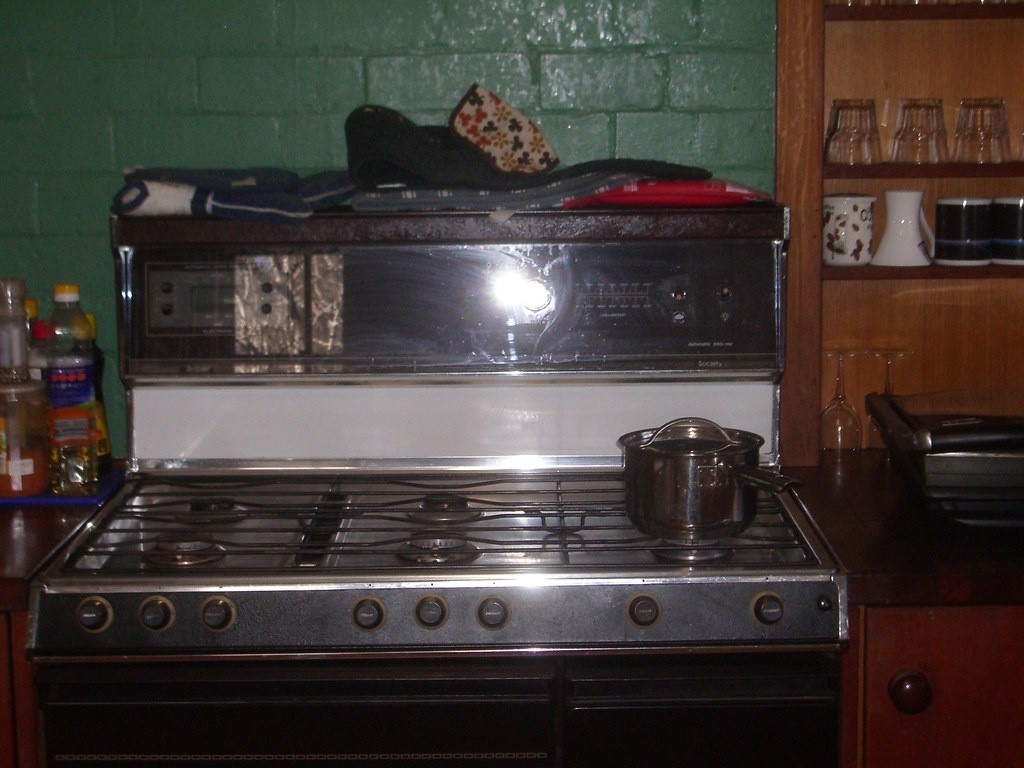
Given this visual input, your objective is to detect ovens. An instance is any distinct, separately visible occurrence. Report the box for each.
[30,660,853,768]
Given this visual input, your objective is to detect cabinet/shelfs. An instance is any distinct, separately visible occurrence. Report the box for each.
[840,592,1024,767]
[777,1,1024,469]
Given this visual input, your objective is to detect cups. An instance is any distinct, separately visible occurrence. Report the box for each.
[890,98,949,165]
[933,199,992,266]
[823,195,876,267]
[827,98,882,166]
[952,97,1014,165]
[992,199,1024,266]
[872,189,936,267]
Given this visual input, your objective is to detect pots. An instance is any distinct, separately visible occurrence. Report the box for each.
[615,417,799,544]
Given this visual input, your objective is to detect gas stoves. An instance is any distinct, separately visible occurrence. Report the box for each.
[24,202,852,657]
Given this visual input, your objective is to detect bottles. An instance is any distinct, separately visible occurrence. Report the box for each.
[0,278,110,498]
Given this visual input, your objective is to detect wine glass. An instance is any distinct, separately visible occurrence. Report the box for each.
[863,349,914,474]
[819,349,866,474]
